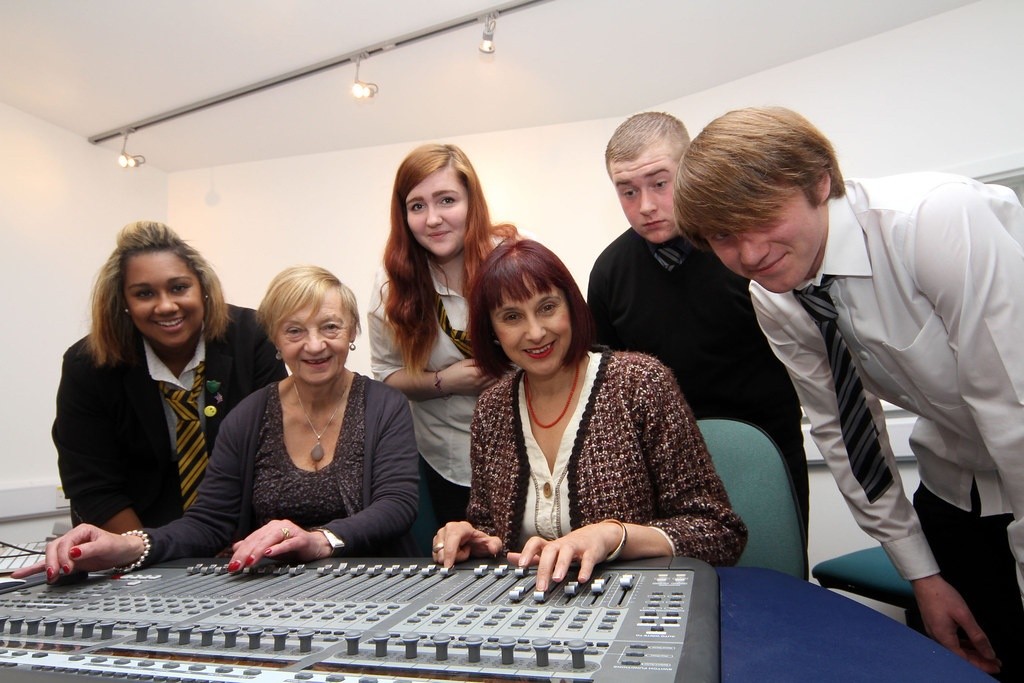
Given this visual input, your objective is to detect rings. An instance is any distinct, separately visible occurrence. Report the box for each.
[433,542,444,553]
[281,527,290,540]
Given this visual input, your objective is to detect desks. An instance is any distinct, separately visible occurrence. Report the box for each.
[716,566,999,683]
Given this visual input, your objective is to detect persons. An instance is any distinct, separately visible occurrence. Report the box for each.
[432,238,748,591]
[367,143,522,524]
[587,112,808,549]
[11,263,420,585]
[672,104,1024,683]
[52,221,289,536]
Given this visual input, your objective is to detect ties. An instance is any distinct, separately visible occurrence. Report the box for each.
[159,361,210,513]
[434,292,474,359]
[793,274,895,505]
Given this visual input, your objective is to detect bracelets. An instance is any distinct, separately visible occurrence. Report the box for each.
[599,519,627,562]
[112,529,151,574]
[435,370,452,401]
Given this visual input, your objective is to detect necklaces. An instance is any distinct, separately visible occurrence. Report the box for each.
[526,359,579,428]
[294,376,349,462]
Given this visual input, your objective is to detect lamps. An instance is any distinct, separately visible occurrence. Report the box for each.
[118,127,145,168]
[477,10,500,54]
[350,51,378,99]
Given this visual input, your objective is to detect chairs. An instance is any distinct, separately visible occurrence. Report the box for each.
[811,545,925,633]
[693,418,808,582]
